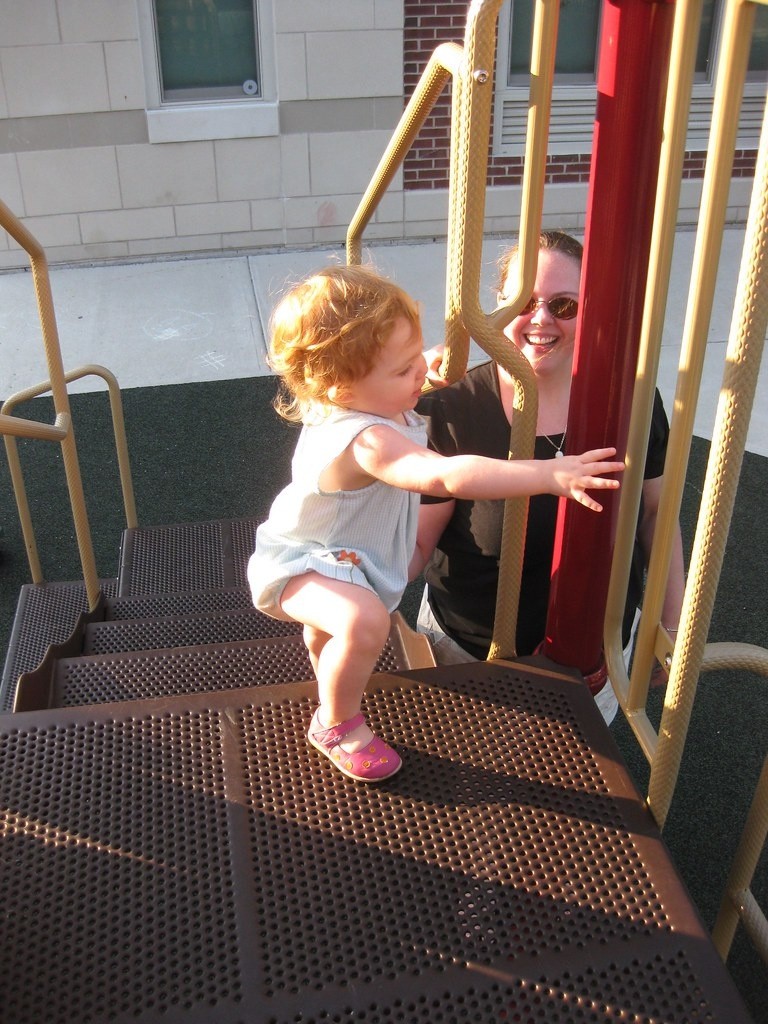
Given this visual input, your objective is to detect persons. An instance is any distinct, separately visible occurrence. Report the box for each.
[407,231,683,726]
[244,265,625,782]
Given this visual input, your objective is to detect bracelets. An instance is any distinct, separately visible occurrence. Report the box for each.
[664,628,680,633]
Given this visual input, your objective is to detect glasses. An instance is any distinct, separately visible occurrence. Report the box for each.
[501,296,578,320]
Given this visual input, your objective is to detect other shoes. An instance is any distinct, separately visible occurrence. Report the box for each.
[308,707,402,783]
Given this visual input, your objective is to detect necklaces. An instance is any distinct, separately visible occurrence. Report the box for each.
[502,370,569,459]
